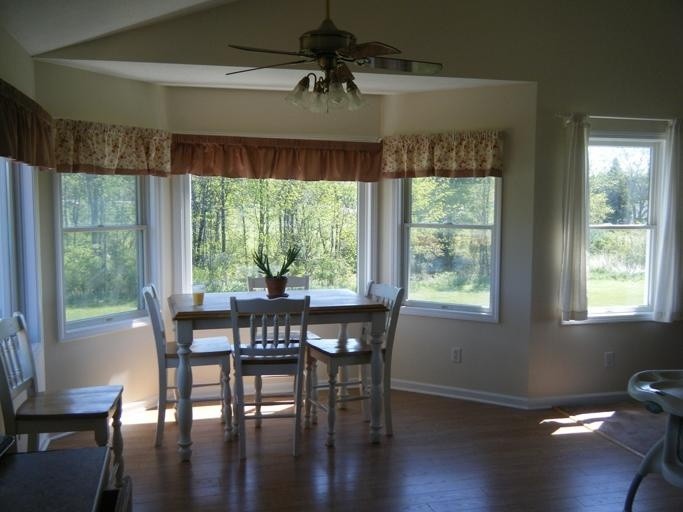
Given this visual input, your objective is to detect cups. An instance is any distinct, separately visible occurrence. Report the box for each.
[192,284,204,305]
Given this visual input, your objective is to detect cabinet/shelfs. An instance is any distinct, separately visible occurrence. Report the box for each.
[0,446,132,512]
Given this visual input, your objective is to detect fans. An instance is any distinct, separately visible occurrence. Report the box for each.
[225,0,442,76]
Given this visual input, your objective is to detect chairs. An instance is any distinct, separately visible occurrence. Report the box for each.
[624,369,683,512]
[0,311,124,488]
[142,283,233,448]
[229,275,318,457]
[305,280,405,447]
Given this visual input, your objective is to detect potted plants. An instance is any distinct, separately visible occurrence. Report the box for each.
[251,244,301,299]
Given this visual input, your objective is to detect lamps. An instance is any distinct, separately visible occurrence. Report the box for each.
[284,52,369,115]
